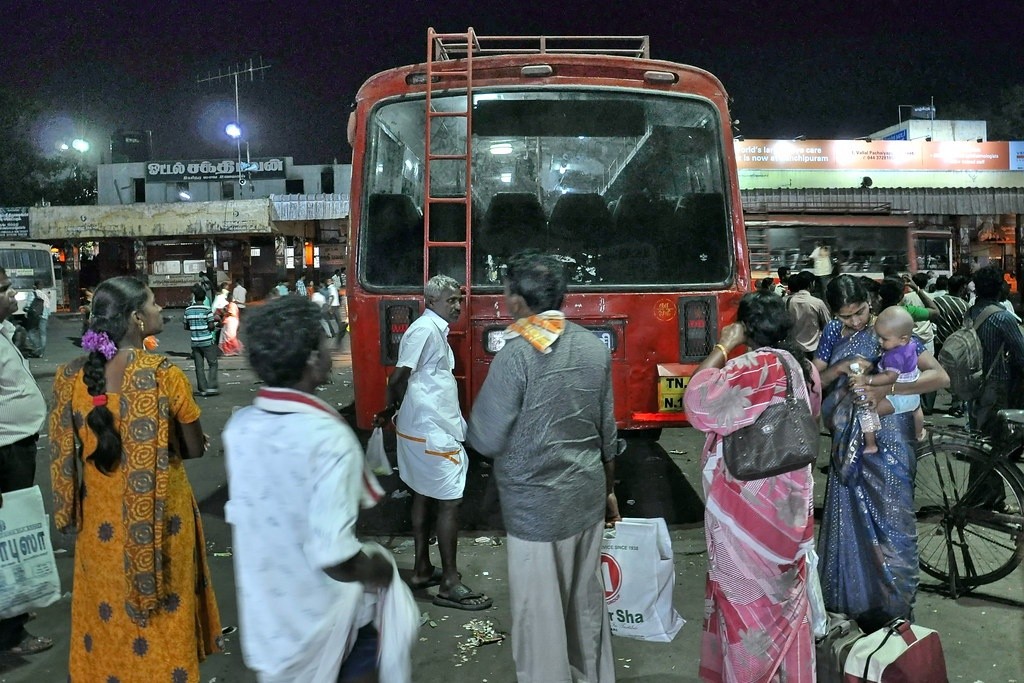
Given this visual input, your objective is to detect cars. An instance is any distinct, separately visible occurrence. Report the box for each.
[862,256,907,272]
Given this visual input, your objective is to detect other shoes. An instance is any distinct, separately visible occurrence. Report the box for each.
[947,406,963,418]
[997,505,1019,512]
[200,388,219,396]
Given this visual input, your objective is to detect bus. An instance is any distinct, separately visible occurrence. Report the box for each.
[911,230,953,278]
[743,214,915,294]
[0,241,57,316]
[347,27,751,441]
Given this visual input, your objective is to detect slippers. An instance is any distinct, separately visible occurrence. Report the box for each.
[27,611,36,623]
[432,591,493,610]
[0,634,53,656]
[406,565,460,590]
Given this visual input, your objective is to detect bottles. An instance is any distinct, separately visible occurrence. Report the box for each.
[850,363,882,433]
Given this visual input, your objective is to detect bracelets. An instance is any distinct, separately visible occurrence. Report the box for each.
[917,285,919,295]
[868,374,872,384]
[713,344,728,366]
[891,383,896,396]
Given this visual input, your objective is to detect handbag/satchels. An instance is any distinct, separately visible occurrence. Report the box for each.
[364,427,393,475]
[844,619,949,683]
[722,349,818,481]
[600,517,687,642]
[0,485,62,618]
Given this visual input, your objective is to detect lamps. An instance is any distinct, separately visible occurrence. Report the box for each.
[853,138,872,143]
[909,135,932,142]
[793,135,806,141]
[967,136,982,143]
[732,135,745,142]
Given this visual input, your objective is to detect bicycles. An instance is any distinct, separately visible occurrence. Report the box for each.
[915,409,1024,600]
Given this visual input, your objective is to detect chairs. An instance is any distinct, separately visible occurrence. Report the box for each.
[364,192,728,287]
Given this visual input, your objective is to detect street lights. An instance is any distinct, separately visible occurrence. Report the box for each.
[226,124,255,199]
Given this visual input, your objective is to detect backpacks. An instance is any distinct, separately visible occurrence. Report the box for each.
[27,292,44,321]
[814,612,866,683]
[937,304,1005,402]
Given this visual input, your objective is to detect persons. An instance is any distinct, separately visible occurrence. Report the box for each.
[274,267,350,351]
[810,274,952,631]
[24,279,50,358]
[758,243,1024,516]
[466,243,623,683]
[223,291,392,683]
[0,264,47,508]
[376,267,494,610]
[183,285,220,397]
[855,304,926,455]
[194,268,247,357]
[681,286,822,683]
[79,282,92,341]
[44,273,223,683]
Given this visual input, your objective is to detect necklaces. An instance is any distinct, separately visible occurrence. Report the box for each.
[840,314,873,341]
